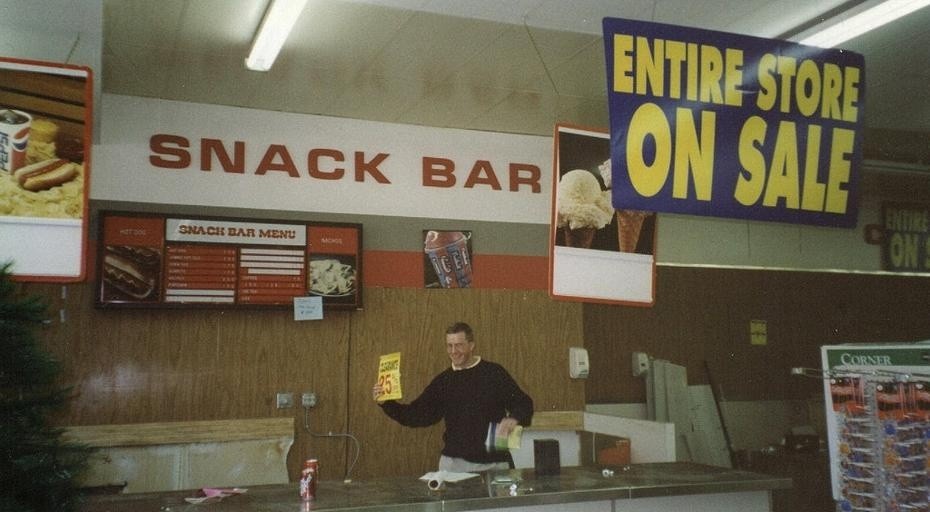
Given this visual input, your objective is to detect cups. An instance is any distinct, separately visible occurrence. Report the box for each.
[0,110,31,173]
[426,233,472,288]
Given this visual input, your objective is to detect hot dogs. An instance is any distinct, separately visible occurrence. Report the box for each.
[14,158,76,191]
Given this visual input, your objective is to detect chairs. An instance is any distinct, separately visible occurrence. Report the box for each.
[508,430,579,469]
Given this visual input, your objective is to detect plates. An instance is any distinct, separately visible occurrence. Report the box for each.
[306,266,357,298]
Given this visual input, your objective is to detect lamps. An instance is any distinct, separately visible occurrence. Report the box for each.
[786,0,930,48]
[245,0,306,72]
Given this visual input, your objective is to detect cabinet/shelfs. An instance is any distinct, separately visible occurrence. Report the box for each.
[36,416,296,496]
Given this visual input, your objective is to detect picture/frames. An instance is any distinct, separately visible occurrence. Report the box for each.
[95,210,363,312]
[549,123,659,308]
[0,57,93,285]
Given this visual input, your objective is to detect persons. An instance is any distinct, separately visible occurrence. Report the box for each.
[371,320,534,477]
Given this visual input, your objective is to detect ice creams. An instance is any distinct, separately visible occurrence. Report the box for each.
[598,159,656,253]
[556,169,615,248]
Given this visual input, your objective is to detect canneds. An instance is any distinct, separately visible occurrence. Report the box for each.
[298,468,315,498]
[303,460,318,490]
